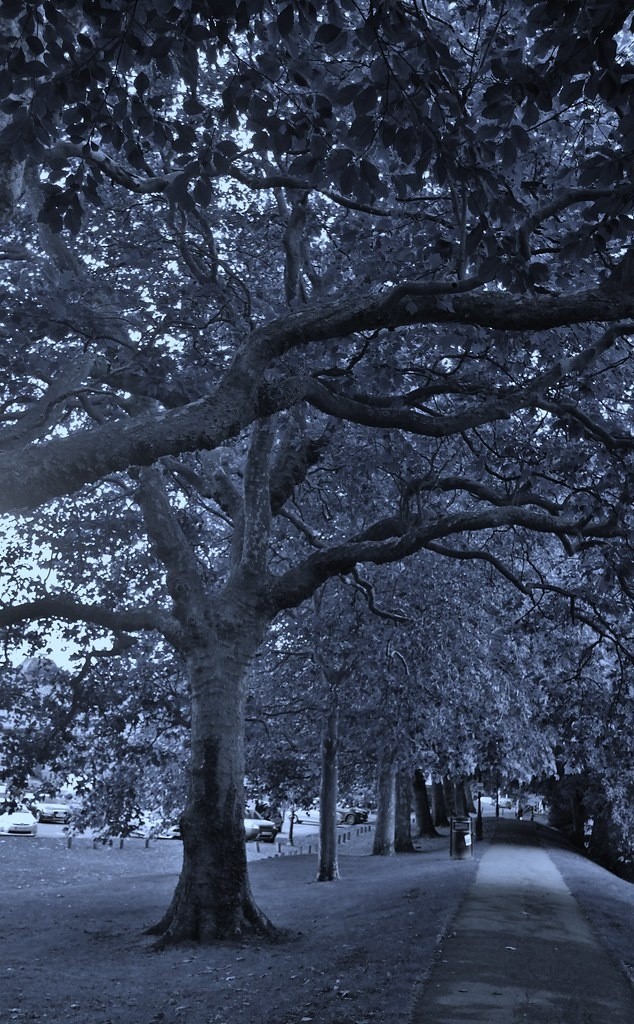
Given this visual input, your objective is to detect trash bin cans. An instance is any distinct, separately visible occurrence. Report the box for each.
[450,816,473,860]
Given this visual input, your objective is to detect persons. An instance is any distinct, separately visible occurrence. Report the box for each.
[583,814,595,848]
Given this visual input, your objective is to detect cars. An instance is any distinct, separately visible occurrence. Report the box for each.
[243,809,278,842]
[287,798,344,826]
[266,809,285,833]
[335,800,370,824]
[0,791,83,837]
